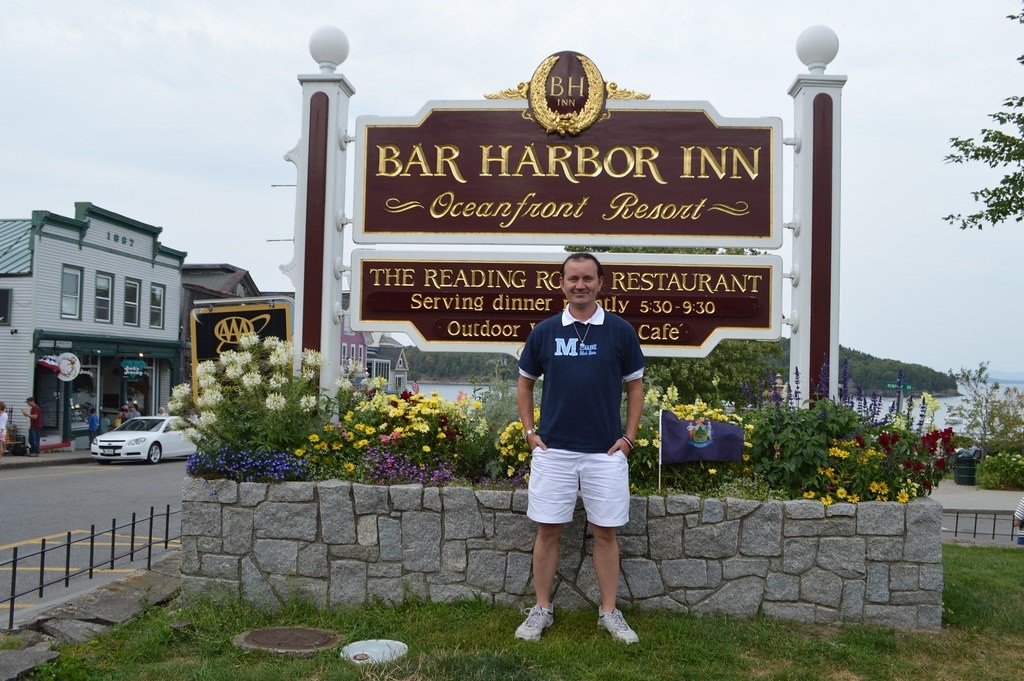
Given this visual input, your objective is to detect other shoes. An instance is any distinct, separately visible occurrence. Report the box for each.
[28,453,39,457]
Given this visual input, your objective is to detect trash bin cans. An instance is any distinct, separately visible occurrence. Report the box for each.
[954,448,983,486]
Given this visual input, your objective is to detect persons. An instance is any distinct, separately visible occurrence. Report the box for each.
[0,404,8,457]
[514,253,645,646]
[23,397,42,457]
[99,412,111,435]
[113,403,141,429]
[85,408,98,450]
[1014,497,1024,544]
[190,410,199,423]
[156,407,169,416]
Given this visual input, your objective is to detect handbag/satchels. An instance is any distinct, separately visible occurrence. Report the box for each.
[113,412,121,427]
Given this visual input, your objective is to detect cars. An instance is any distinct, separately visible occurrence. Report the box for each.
[91,416,198,463]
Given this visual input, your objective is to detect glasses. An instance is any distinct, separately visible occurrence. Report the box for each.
[131,407,135,409]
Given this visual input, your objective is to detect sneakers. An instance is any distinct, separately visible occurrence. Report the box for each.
[515,603,553,642]
[597,606,639,645]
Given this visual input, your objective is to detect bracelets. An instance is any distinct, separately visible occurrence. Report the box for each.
[524,428,536,442]
[623,435,635,450]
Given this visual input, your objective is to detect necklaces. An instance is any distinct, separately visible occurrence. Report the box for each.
[573,322,591,350]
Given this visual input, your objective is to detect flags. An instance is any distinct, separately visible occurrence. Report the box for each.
[659,408,744,465]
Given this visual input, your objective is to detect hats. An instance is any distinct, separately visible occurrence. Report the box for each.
[25,397,34,402]
[122,405,128,409]
